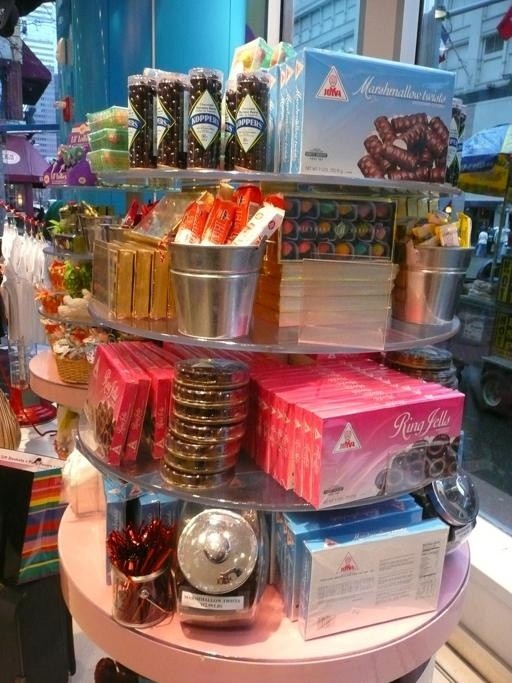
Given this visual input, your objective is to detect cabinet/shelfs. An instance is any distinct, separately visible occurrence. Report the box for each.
[23,151,478,682]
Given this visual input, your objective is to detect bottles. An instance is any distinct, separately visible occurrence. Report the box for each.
[111,502,267,630]
[128,68,270,172]
[411,473,479,555]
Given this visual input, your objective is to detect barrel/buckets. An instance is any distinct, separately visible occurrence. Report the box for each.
[166,236,276,340]
[394,238,472,324]
